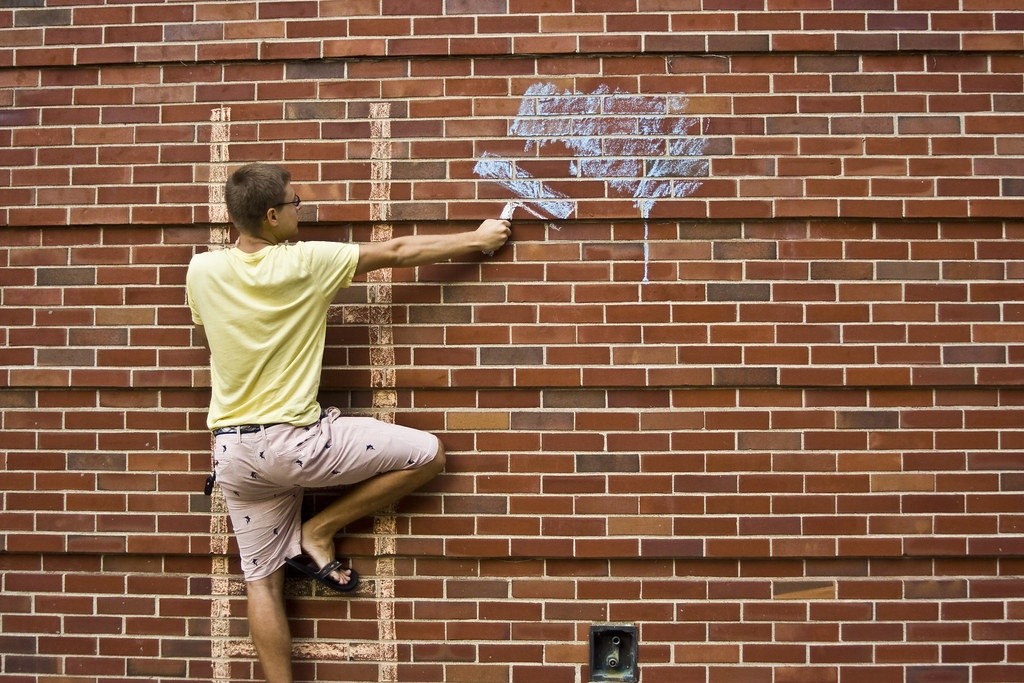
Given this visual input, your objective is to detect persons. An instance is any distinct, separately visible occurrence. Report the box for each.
[186,163,512,683]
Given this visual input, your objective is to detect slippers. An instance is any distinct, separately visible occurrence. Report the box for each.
[284,550,358,591]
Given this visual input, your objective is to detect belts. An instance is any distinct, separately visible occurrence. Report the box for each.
[213,423,279,436]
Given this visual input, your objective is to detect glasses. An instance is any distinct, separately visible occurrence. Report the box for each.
[264,195,301,223]
